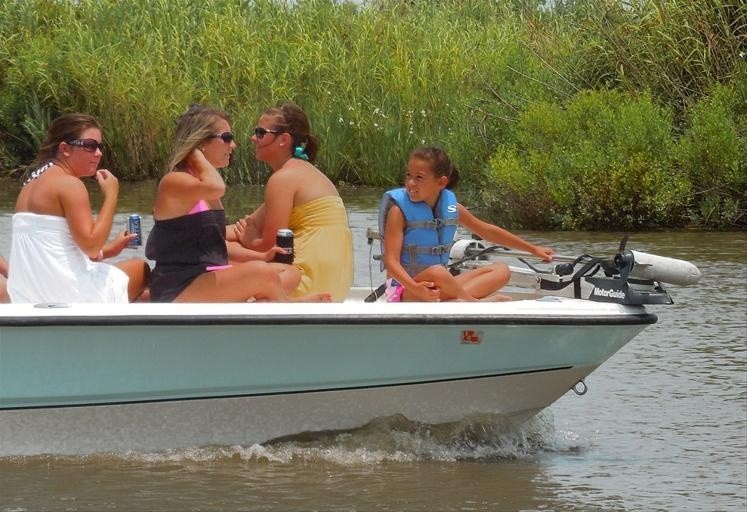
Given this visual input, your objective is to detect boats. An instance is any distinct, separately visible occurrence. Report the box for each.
[1,232,703,455]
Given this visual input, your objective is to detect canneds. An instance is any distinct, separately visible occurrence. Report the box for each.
[276,229,294,263]
[126,215,142,245]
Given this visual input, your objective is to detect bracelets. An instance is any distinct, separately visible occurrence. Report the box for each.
[97,250,103,262]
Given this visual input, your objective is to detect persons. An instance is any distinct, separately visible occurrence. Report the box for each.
[375,146,553,303]
[224,100,354,302]
[144,107,334,303]
[5,112,149,304]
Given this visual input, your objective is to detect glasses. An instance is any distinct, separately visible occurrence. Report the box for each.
[206,132,234,142]
[66,139,104,152]
[255,127,281,138]
